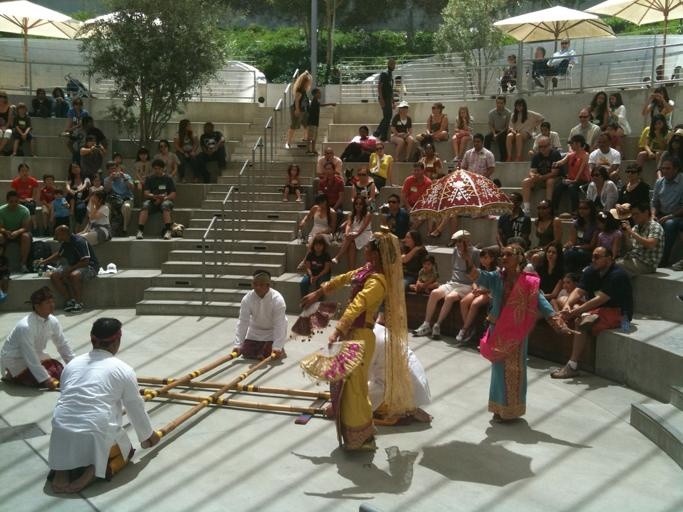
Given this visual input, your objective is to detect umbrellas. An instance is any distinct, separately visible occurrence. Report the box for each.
[493,4,618,55]
[79,11,164,38]
[585,0,683,76]
[408,161,515,249]
[0,0,82,94]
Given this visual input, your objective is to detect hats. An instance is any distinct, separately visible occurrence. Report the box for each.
[396,100,410,108]
[609,202,633,220]
[565,134,587,145]
[107,262,118,274]
[450,228,472,241]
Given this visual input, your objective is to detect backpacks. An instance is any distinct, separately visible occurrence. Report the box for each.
[27,240,53,273]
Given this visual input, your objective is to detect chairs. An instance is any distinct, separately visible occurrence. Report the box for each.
[543,64,576,93]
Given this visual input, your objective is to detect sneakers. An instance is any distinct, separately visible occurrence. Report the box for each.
[463,328,474,342]
[136,231,143,239]
[64,298,76,312]
[164,230,172,240]
[69,302,86,313]
[456,328,465,341]
[432,323,441,339]
[21,263,30,273]
[412,322,433,336]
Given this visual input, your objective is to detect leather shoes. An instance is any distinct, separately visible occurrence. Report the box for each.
[551,363,580,378]
[579,312,600,330]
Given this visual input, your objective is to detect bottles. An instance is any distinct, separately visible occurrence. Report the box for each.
[622,311,629,332]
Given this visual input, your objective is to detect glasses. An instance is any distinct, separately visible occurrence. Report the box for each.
[536,144,550,149]
[578,205,591,210]
[8,199,19,204]
[624,168,640,174]
[388,200,398,204]
[498,251,517,257]
[536,204,551,210]
[560,41,571,45]
[591,253,609,259]
[375,147,384,150]
[355,202,363,206]
[357,173,367,176]
[590,173,602,177]
[579,114,590,118]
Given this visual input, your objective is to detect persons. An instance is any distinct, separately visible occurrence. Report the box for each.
[563,200,598,271]
[456,237,585,423]
[0,191,32,274]
[340,125,379,162]
[300,233,333,300]
[393,76,407,109]
[317,146,344,186]
[373,57,396,142]
[389,101,415,162]
[587,133,622,181]
[612,200,665,279]
[401,230,430,292]
[642,63,675,87]
[231,270,290,359]
[39,173,59,236]
[568,107,602,157]
[78,135,106,174]
[550,271,586,319]
[50,188,72,234]
[0,286,75,390]
[654,125,683,179]
[283,70,313,151]
[350,166,377,204]
[77,190,113,247]
[35,224,100,314]
[457,133,495,178]
[331,194,375,271]
[550,245,636,378]
[416,102,449,144]
[326,320,435,425]
[73,115,107,167]
[456,249,502,343]
[304,88,336,156]
[383,194,409,240]
[279,164,306,202]
[400,161,433,236]
[111,152,129,174]
[367,143,394,189]
[591,210,624,262]
[13,105,37,157]
[173,118,201,184]
[297,232,398,451]
[82,173,104,220]
[639,86,674,151]
[483,95,512,161]
[524,199,562,268]
[0,91,13,156]
[10,163,39,216]
[409,255,440,295]
[132,148,151,183]
[64,161,90,233]
[497,191,532,252]
[649,154,683,267]
[535,241,570,300]
[551,135,590,216]
[522,135,564,217]
[417,143,444,179]
[636,114,674,173]
[588,91,609,131]
[585,166,619,216]
[63,96,88,154]
[605,93,632,144]
[533,37,578,89]
[430,210,460,247]
[614,162,650,215]
[412,230,481,340]
[505,97,544,162]
[52,87,70,118]
[101,159,136,238]
[196,120,226,183]
[528,121,563,161]
[150,139,180,181]
[298,193,336,273]
[135,158,177,240]
[526,46,548,81]
[449,105,474,163]
[316,162,345,209]
[27,88,53,118]
[500,53,518,94]
[48,317,159,493]
